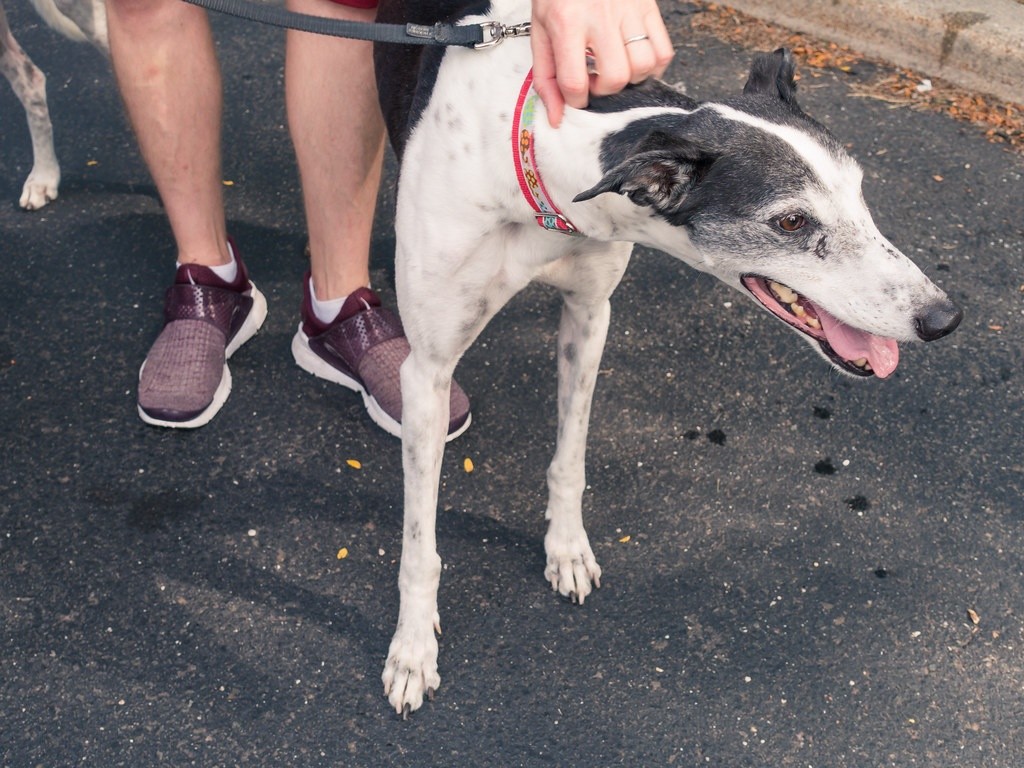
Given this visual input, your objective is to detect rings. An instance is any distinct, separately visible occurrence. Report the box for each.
[625,35,650,45]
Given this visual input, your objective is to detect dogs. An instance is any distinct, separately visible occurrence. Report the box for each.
[368,0,967,721]
[0,0,112,213]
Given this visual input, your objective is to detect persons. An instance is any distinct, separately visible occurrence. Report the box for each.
[104,0,675,444]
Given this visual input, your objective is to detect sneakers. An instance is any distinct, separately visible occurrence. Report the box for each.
[138,234,270,429]
[292,268,473,442]
